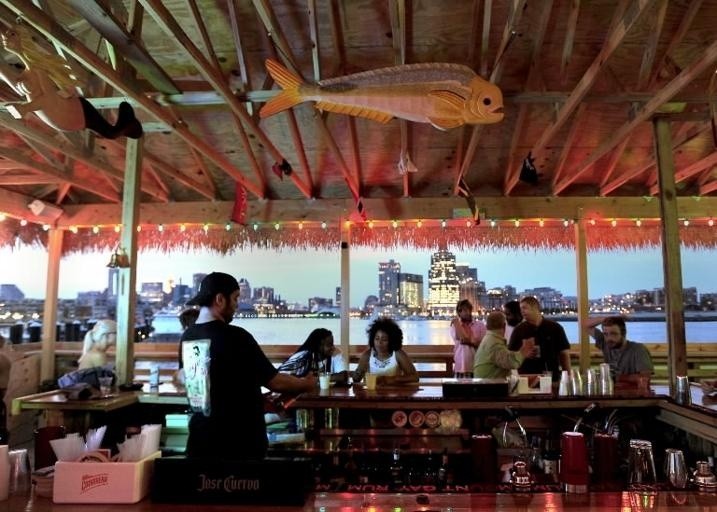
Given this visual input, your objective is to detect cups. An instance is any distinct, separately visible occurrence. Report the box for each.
[98,377,113,397]
[365,372,378,389]
[318,372,331,390]
[557,362,615,398]
[533,344,541,358]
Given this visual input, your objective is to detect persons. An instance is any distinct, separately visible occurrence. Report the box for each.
[271,327,348,400]
[77,318,116,378]
[178,272,318,505]
[582,313,655,385]
[449,299,486,377]
[471,311,535,379]
[350,318,420,390]
[506,295,571,385]
[504,300,523,325]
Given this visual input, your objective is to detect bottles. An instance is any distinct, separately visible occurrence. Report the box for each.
[626,438,656,496]
[389,447,405,488]
[436,451,454,492]
[664,446,689,491]
[510,461,535,492]
[9,448,32,498]
[690,459,716,494]
[676,376,692,396]
[560,432,590,494]
[150,365,161,387]
[531,436,557,474]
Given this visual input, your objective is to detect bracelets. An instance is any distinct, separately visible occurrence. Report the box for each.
[618,373,629,382]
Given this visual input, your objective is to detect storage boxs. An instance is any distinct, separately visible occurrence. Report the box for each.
[52,450,161,504]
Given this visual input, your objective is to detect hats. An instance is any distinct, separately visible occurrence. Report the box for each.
[186,273,237,307]
